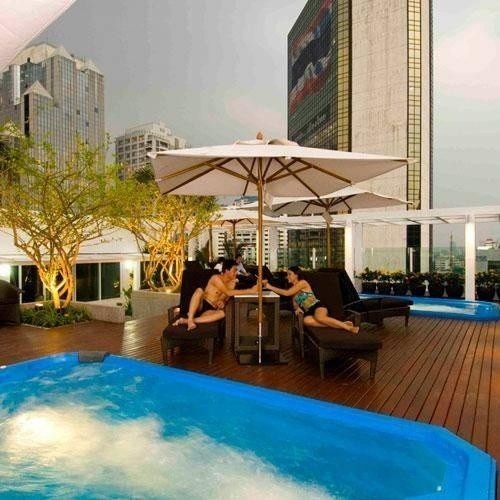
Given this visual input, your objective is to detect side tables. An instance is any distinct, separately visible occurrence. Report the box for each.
[230,290,281,365]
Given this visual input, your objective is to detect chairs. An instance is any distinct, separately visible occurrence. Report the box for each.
[292,271,383,381]
[161,268,226,366]
[182,260,292,310]
[318,268,414,328]
[0,280,27,328]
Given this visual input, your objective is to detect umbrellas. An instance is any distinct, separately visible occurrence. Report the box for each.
[235,185,413,268]
[208,209,288,258]
[145,131,416,364]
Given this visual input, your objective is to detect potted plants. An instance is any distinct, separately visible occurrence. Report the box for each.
[357,269,497,299]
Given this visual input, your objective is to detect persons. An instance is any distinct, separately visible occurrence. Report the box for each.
[262,265,360,335]
[213,256,225,273]
[172,259,258,332]
[234,253,248,276]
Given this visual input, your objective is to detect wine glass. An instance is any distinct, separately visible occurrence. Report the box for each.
[262,280,268,289]
